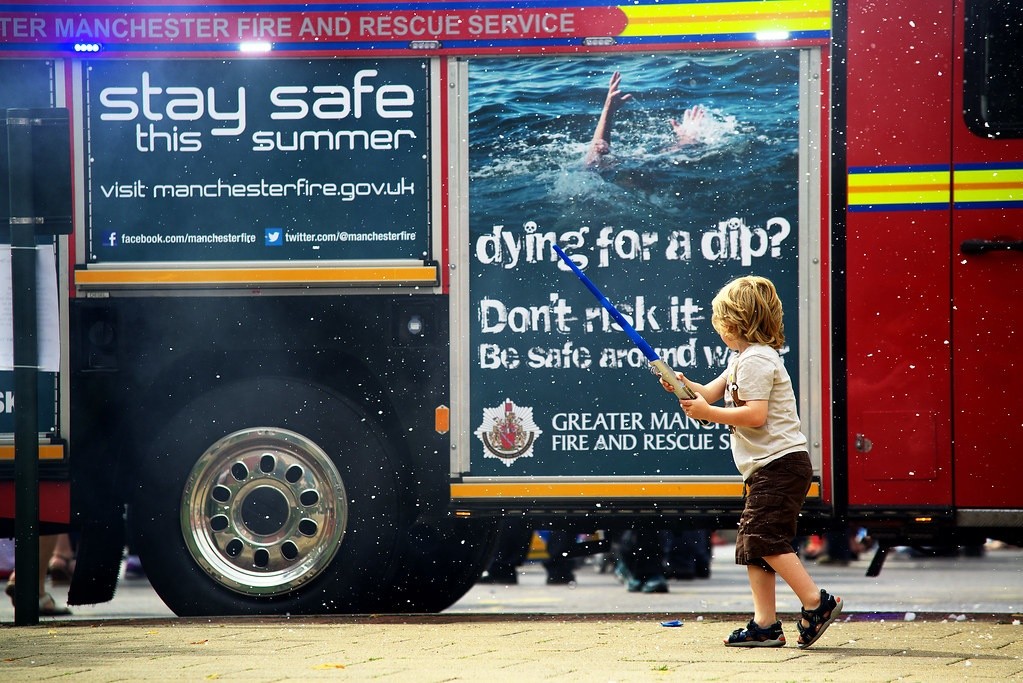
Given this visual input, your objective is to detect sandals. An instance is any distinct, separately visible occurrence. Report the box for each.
[796,589,843,649]
[5,567,55,612]
[47,553,72,580]
[724,618,786,647]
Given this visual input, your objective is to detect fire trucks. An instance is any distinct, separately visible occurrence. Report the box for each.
[0,0,1023,630]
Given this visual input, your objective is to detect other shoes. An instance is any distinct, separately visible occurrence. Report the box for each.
[629,575,669,592]
[547,567,575,584]
[481,570,517,583]
[123,554,145,579]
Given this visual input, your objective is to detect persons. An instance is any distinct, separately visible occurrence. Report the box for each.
[660,276,843,649]
[480,523,667,592]
[588,71,706,163]
[6,535,75,617]
